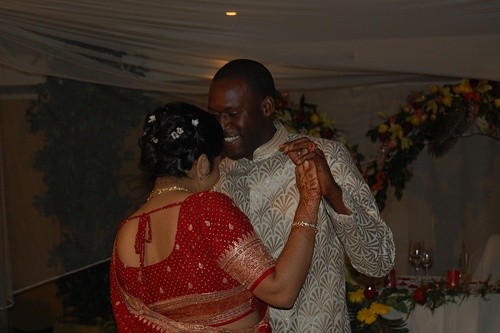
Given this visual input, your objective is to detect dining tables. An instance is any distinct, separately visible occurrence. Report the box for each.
[379,281,500,333]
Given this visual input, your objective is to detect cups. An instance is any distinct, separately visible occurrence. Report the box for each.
[447,268,460,289]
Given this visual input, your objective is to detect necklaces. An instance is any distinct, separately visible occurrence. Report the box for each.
[147,186,192,201]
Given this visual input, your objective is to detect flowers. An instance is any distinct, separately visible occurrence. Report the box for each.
[345,268,500,333]
[275,78,500,212]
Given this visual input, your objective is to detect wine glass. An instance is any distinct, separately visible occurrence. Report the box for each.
[408,239,424,276]
[415,249,432,277]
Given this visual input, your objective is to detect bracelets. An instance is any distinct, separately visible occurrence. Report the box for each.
[291,220,318,234]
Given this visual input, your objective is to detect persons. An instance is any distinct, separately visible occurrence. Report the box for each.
[208,58,396,333]
[109,100,323,333]
